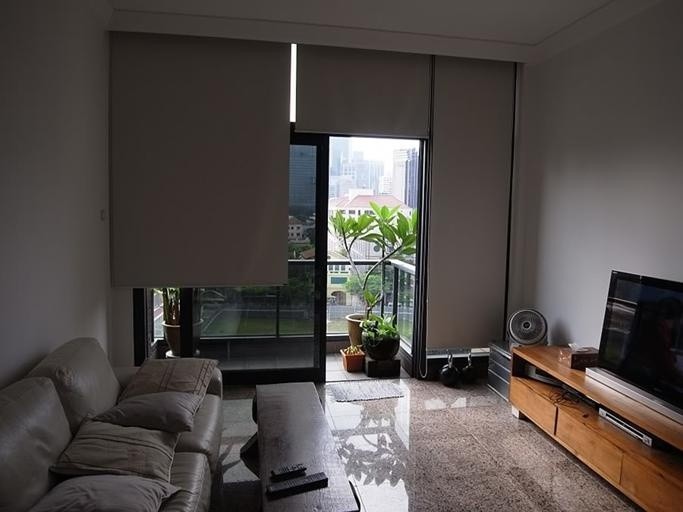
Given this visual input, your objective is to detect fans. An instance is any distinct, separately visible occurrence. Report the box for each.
[507,307,546,351]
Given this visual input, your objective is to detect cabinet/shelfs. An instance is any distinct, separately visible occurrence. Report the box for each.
[507,346,683,512]
[485,340,512,402]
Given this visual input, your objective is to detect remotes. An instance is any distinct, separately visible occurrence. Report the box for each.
[270,462,307,479]
[266,471,329,498]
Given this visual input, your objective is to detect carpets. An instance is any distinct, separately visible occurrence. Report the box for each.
[330,380,405,402]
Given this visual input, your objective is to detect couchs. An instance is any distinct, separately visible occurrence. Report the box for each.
[1,337,223,512]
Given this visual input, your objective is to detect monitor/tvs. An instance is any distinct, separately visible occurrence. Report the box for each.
[596,270,683,413]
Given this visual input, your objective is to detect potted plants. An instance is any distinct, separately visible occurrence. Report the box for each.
[359,315,400,361]
[339,345,364,372]
[156,287,203,356]
[326,201,417,347]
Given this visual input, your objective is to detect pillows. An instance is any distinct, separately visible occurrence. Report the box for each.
[93,391,204,433]
[118,357,217,402]
[26,473,181,512]
[48,414,182,485]
